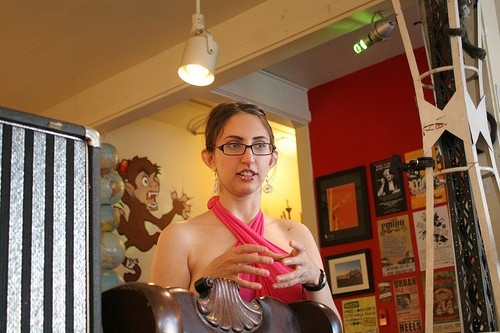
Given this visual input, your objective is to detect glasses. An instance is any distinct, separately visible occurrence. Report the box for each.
[209,142,276,156]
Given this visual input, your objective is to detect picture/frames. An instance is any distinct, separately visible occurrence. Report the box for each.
[324,249,374,298]
[314,166,373,247]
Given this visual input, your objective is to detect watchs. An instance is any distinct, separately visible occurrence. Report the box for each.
[301,269,329,292]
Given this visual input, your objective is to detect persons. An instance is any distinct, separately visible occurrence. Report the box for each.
[377,169,401,197]
[149,102,343,333]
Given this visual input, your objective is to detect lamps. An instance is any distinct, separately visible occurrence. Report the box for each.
[177,0,218,87]
[354,12,395,54]
